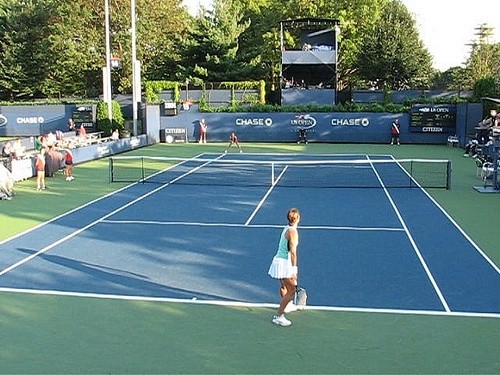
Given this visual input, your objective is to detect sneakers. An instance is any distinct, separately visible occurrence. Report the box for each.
[65,177,72,182]
[70,176,75,179]
[284,301,297,313]
[272,314,292,326]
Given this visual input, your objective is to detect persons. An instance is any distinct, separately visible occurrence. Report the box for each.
[198,119,208,143]
[112,129,119,141]
[297,125,308,145]
[225,132,242,153]
[65,144,75,181]
[34,148,45,191]
[463,109,500,159]
[67,118,75,131]
[391,119,401,145]
[268,208,301,327]
[35,129,64,150]
[13,138,26,160]
[2,141,16,161]
[79,124,86,135]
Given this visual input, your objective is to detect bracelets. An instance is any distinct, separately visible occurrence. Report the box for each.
[292,266,298,274]
